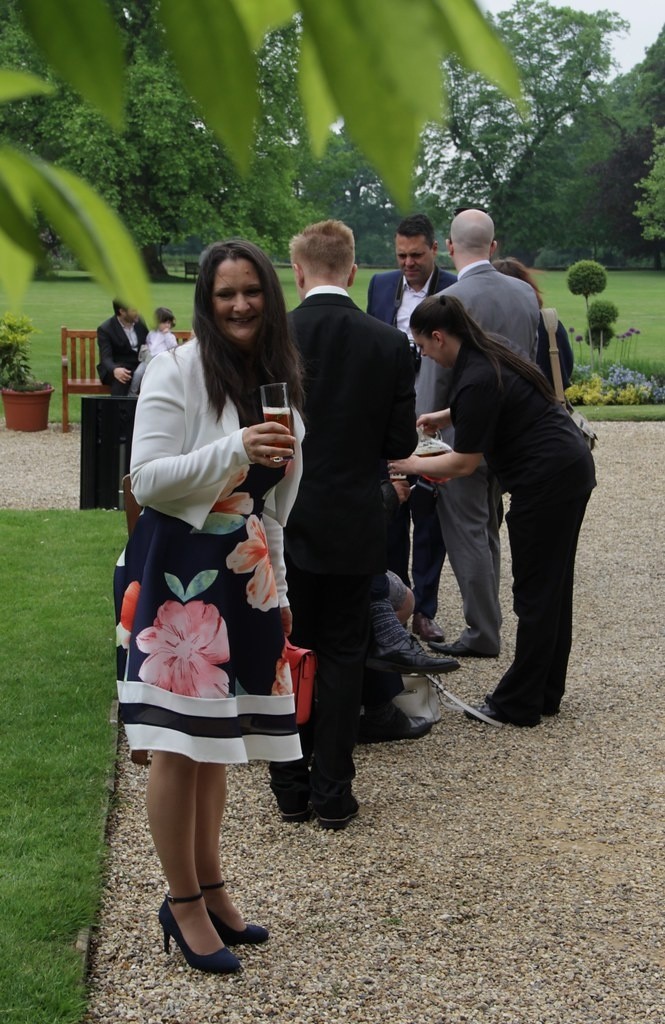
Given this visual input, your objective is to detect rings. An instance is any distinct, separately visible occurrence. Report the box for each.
[390,463,393,468]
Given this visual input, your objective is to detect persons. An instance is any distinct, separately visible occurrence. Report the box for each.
[113,239,305,974]
[265,221,418,829]
[98,209,598,741]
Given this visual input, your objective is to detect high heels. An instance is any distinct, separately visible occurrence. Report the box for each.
[200,880,269,946]
[159,889,241,973]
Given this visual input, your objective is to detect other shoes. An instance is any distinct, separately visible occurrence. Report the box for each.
[274,789,359,830]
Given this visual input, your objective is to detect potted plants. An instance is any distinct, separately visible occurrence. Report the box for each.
[0,309,55,433]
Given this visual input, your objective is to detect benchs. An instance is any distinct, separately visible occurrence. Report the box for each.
[61,326,195,433]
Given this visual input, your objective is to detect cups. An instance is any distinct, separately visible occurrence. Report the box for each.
[260,382,295,459]
[391,472,408,483]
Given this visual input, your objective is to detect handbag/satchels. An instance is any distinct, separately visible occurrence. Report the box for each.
[392,672,503,729]
[556,396,598,451]
[283,635,318,726]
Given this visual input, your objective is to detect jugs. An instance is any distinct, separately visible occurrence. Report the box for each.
[408,425,456,483]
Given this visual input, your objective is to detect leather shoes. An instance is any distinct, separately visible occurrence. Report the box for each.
[365,633,460,677]
[412,612,445,642]
[356,706,433,743]
[464,694,560,731]
[428,638,499,658]
